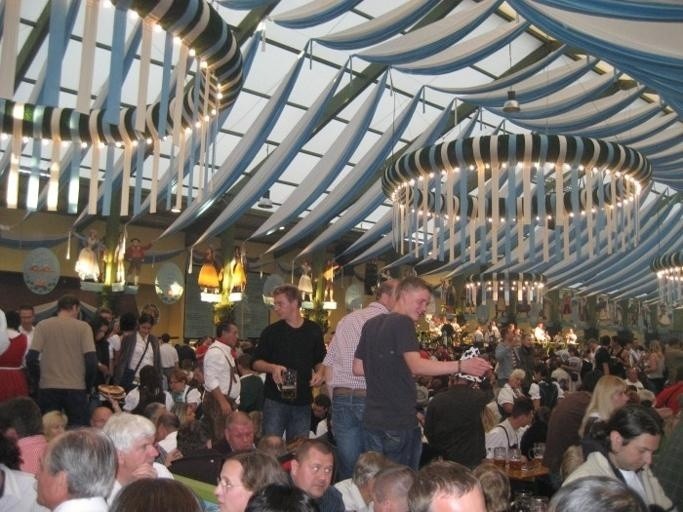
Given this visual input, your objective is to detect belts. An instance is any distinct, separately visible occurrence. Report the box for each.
[333,388,368,400]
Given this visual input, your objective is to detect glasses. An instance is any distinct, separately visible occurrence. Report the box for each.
[214,475,249,494]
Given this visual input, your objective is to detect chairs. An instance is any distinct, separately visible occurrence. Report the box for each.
[165,451,223,486]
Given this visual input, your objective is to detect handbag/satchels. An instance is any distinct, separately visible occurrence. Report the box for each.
[121,368,136,391]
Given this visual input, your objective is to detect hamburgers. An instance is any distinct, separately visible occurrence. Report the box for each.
[98,384,126,399]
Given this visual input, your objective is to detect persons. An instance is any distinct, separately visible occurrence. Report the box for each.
[198,244,248,295]
[298,257,341,303]
[67,223,156,287]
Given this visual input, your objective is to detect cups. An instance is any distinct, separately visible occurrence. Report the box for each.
[493,442,550,512]
[276,368,298,400]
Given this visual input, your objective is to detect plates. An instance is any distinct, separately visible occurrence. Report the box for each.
[102,391,127,399]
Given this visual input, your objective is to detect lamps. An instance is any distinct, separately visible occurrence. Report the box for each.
[0,0,246,219]
[379,27,660,270]
[647,243,683,312]
[459,221,549,308]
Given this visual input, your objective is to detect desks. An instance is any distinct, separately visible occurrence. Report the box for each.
[477,453,550,507]
